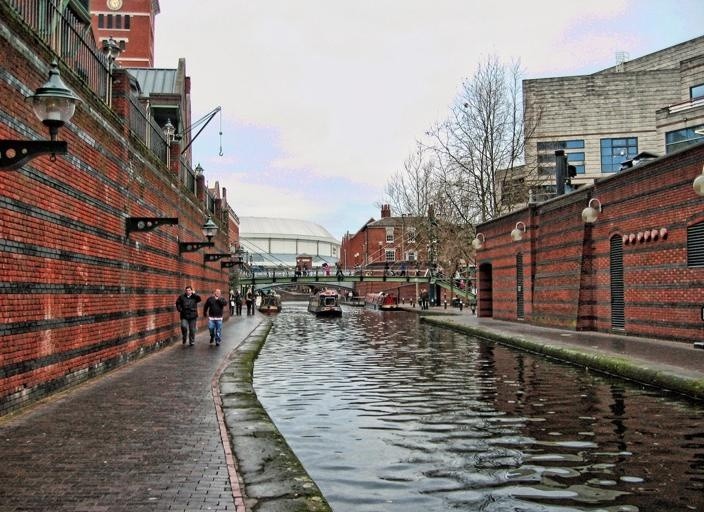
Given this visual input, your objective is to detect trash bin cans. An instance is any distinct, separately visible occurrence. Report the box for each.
[452,298,461,307]
[291,277,297,281]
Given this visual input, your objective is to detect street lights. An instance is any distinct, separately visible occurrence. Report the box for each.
[0,55,82,171]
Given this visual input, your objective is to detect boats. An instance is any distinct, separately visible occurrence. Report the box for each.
[308,287,398,316]
[256,289,281,312]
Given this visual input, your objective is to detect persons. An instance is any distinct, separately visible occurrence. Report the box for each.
[229,284,279,316]
[384,262,390,275]
[418,286,429,309]
[296,281,360,302]
[414,261,421,277]
[203,288,228,347]
[176,286,201,346]
[398,261,406,277]
[450,269,477,296]
[322,262,344,276]
[295,261,309,277]
